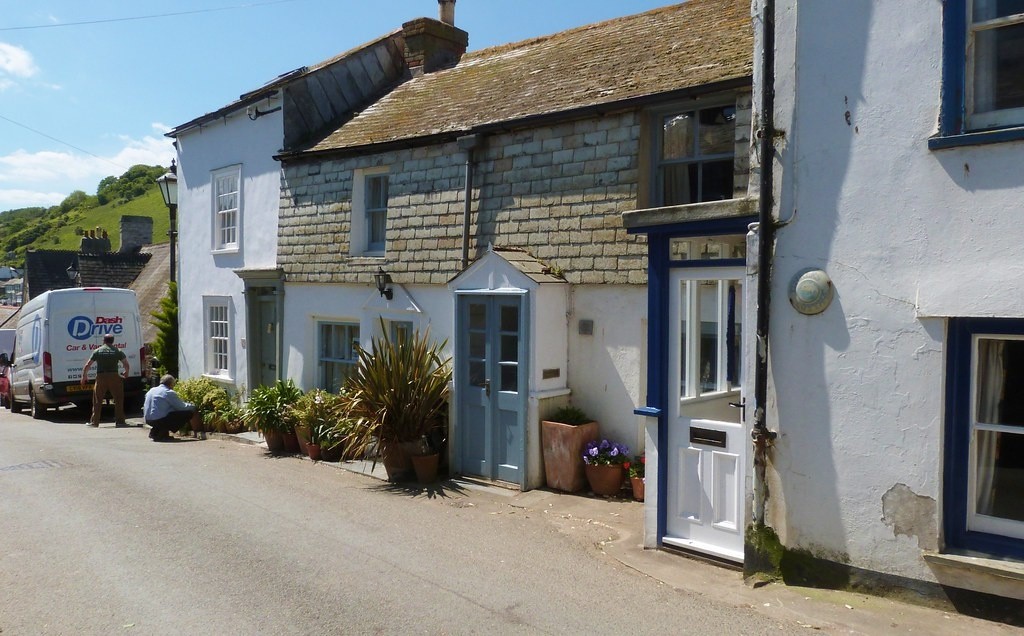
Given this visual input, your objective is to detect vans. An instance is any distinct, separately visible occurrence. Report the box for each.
[0,329,18,375]
[0,284,141,419]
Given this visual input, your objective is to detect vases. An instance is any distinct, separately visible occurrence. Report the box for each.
[630,477,644,501]
[585,463,626,496]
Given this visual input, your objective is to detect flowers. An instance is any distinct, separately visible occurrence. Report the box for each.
[629,446,645,477]
[583,431,631,466]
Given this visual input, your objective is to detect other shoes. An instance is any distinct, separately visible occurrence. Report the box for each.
[86,423,98,427]
[149,432,162,439]
[117,423,130,428]
[154,436,174,442]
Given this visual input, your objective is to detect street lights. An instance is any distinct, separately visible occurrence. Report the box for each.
[155,158,180,299]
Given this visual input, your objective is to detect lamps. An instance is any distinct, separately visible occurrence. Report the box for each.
[373,266,394,301]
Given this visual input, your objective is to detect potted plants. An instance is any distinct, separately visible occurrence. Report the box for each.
[541,405,600,493]
[271,379,305,452]
[412,434,440,483]
[238,382,282,451]
[185,377,211,432]
[222,404,242,434]
[329,387,363,460]
[322,314,452,482]
[299,422,320,459]
[213,399,229,433]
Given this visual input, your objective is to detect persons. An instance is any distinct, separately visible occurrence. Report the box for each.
[143,374,196,441]
[81,334,130,428]
